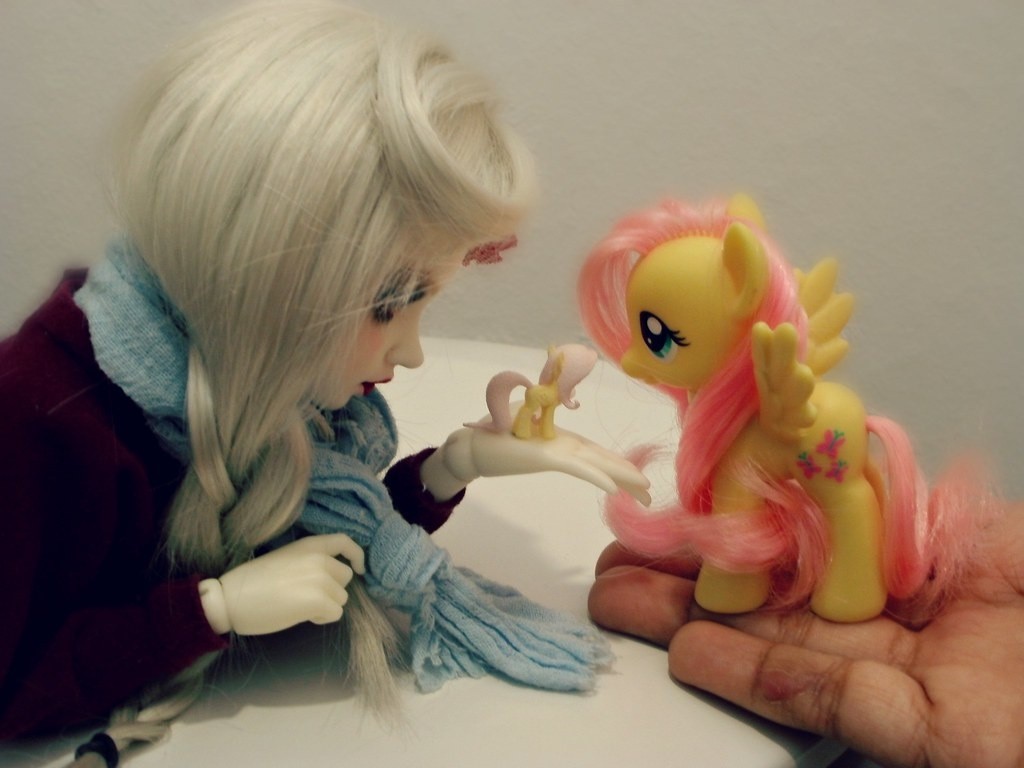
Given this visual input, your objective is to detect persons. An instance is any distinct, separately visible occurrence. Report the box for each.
[0,5,652,762]
[587,502,1024,768]
